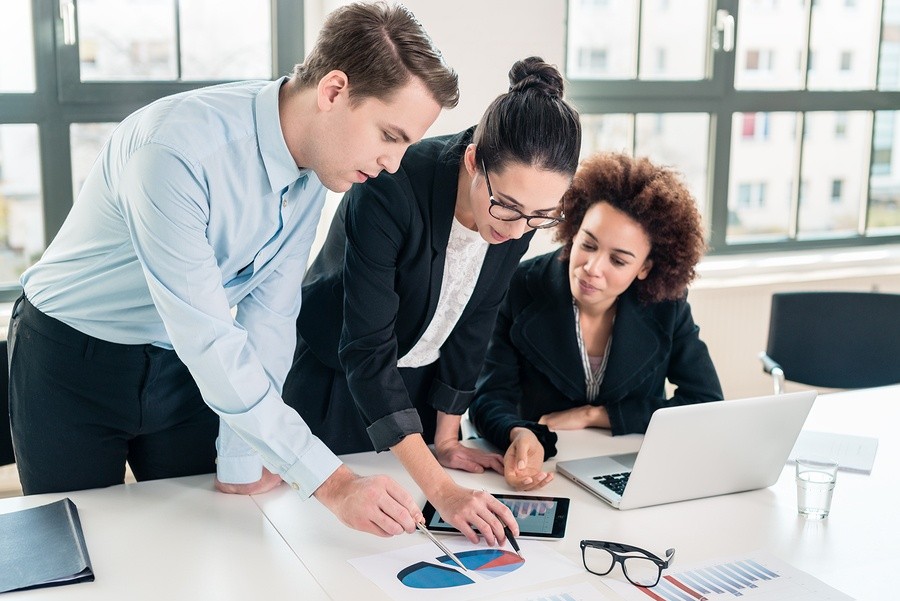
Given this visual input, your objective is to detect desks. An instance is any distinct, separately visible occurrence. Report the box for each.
[0,384,900,601]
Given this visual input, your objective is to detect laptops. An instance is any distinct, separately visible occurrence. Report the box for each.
[554,389,817,511]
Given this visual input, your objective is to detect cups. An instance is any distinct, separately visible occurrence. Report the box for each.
[795,453,841,522]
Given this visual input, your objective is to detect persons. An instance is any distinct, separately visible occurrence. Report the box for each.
[7,0,460,536]
[469,153,725,488]
[281,56,583,545]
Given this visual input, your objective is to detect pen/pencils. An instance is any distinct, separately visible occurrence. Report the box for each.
[415,522,470,575]
[500,521,526,563]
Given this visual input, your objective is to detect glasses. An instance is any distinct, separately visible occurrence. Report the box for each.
[580,540,676,588]
[480,157,568,230]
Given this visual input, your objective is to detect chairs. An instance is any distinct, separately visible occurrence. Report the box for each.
[760,290,900,396]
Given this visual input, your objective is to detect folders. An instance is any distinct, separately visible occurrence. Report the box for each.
[0,499,94,594]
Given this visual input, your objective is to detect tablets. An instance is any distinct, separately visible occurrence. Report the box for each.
[416,493,572,540]
